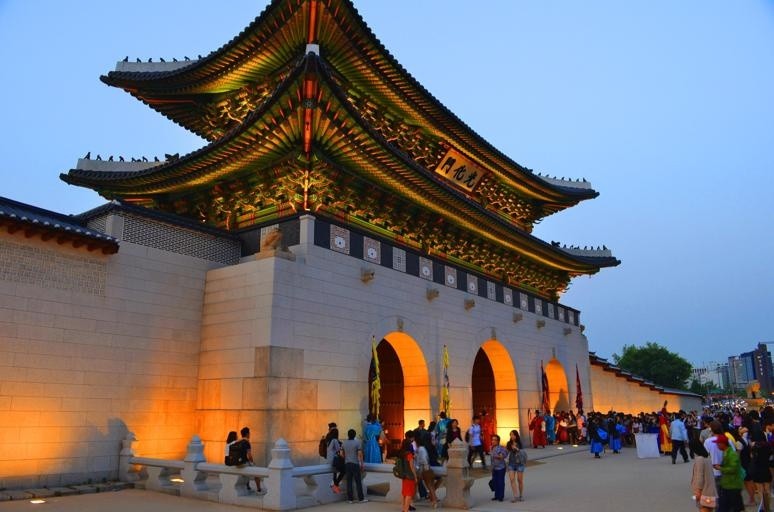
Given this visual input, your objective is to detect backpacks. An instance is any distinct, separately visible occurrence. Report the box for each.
[393,456,407,478]
[229,444,244,464]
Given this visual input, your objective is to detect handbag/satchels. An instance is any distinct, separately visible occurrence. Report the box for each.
[422,469,433,480]
[334,456,343,470]
[700,496,716,508]
[319,439,327,458]
[489,480,495,491]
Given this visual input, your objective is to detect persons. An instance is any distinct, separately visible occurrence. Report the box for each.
[490,434,508,501]
[468,416,486,468]
[399,412,463,512]
[234,426,265,494]
[580,400,671,457]
[669,399,773,512]
[364,415,388,464]
[528,409,577,449]
[506,430,524,502]
[225,431,238,466]
[326,422,368,503]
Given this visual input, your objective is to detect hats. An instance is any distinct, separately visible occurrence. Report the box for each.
[712,436,727,443]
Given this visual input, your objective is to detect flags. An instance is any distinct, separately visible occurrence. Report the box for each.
[368,337,382,415]
[442,347,452,418]
[541,365,549,415]
[576,367,583,415]
